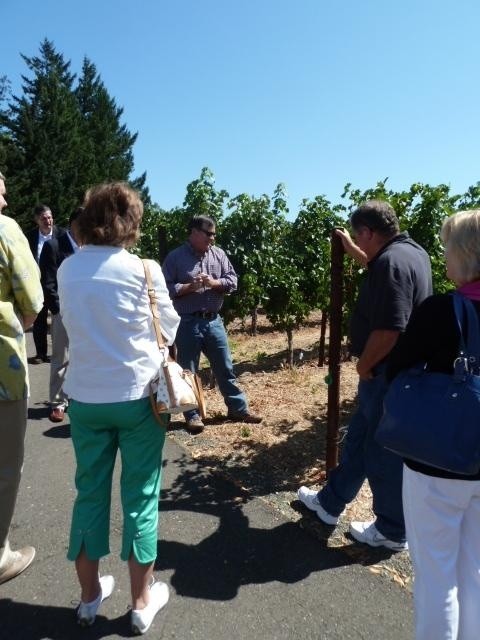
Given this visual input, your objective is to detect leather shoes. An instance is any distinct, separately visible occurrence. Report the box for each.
[48,405,65,423]
[31,355,48,364]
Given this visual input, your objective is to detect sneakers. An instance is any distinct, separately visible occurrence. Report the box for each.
[184,414,205,432]
[75,575,117,629]
[226,410,263,424]
[127,578,171,636]
[296,484,342,528]
[347,519,410,554]
[0,543,36,586]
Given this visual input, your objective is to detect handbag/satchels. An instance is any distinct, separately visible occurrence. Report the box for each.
[154,359,201,416]
[374,288,479,477]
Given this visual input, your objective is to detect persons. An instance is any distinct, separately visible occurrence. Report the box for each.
[1,177,45,581]
[23,205,59,363]
[39,208,82,422]
[296,202,434,551]
[163,216,262,432]
[402,210,480,640]
[56,184,180,635]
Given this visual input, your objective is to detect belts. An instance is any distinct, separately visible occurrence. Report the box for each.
[193,309,216,320]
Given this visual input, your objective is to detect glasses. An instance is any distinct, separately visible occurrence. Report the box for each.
[199,225,217,238]
